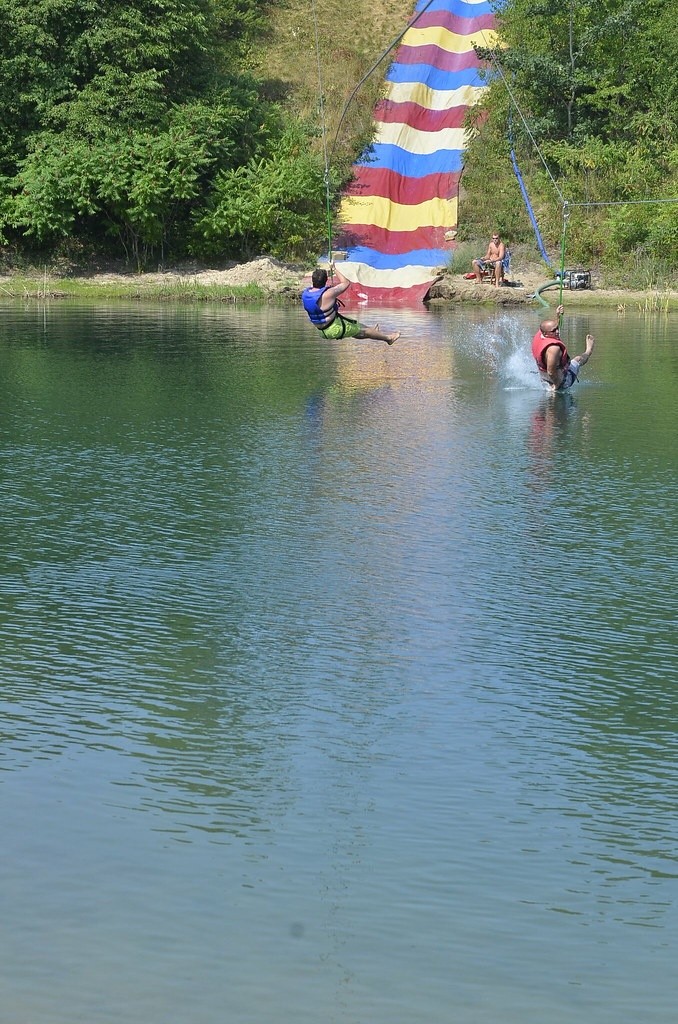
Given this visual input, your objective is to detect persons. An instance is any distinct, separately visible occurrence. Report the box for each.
[302,257,401,345]
[533,304,595,392]
[472,233,506,288]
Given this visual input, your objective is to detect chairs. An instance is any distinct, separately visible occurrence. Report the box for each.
[481,247,510,285]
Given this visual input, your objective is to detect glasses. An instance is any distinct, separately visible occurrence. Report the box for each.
[547,326,558,335]
[492,238,498,239]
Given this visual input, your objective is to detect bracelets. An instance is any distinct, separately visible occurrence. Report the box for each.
[333,268,336,271]
